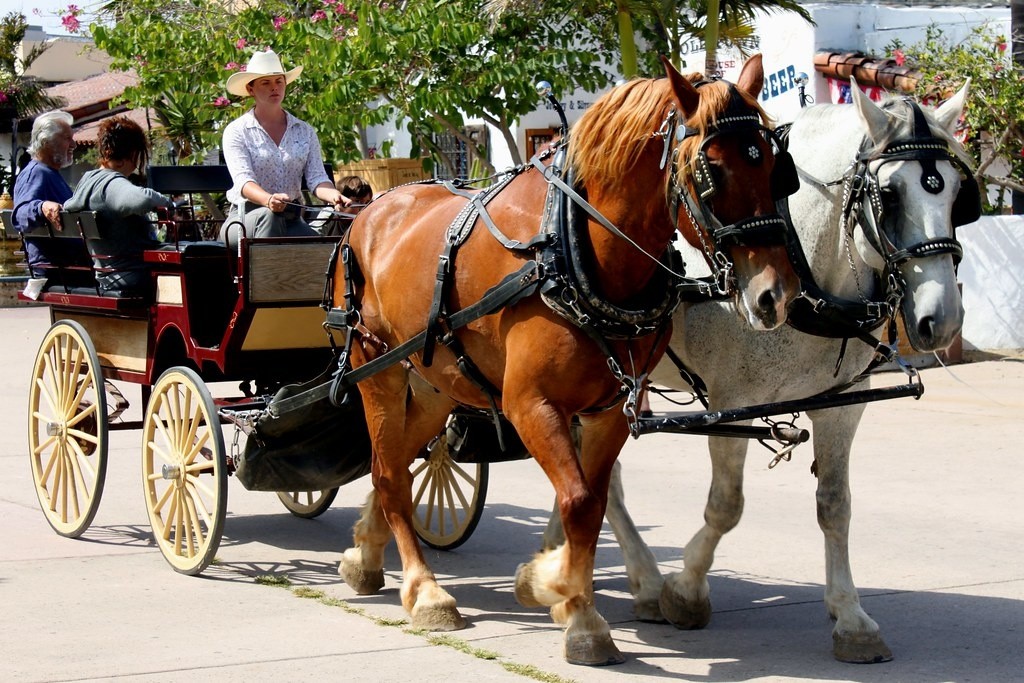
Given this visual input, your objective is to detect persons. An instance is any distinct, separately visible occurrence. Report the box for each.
[10,110,99,289]
[318,175,374,237]
[64,115,175,291]
[219,51,352,253]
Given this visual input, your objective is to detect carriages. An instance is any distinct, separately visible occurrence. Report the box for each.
[0,54,984,666]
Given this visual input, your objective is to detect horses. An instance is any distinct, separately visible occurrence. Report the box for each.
[336,54,971,666]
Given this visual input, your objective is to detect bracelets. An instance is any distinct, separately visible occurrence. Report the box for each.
[266,195,273,209]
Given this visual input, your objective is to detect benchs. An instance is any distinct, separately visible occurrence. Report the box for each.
[7,164,342,379]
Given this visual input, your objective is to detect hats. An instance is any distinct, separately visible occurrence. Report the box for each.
[226,50,304,96]
